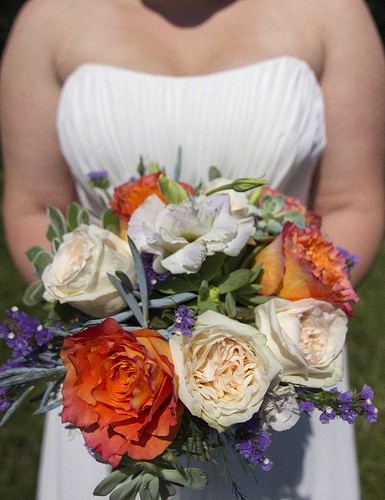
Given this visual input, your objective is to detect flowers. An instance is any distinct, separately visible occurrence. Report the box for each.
[1,146,380,499]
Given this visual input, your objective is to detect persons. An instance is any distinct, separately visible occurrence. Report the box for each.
[1,0,385,499]
